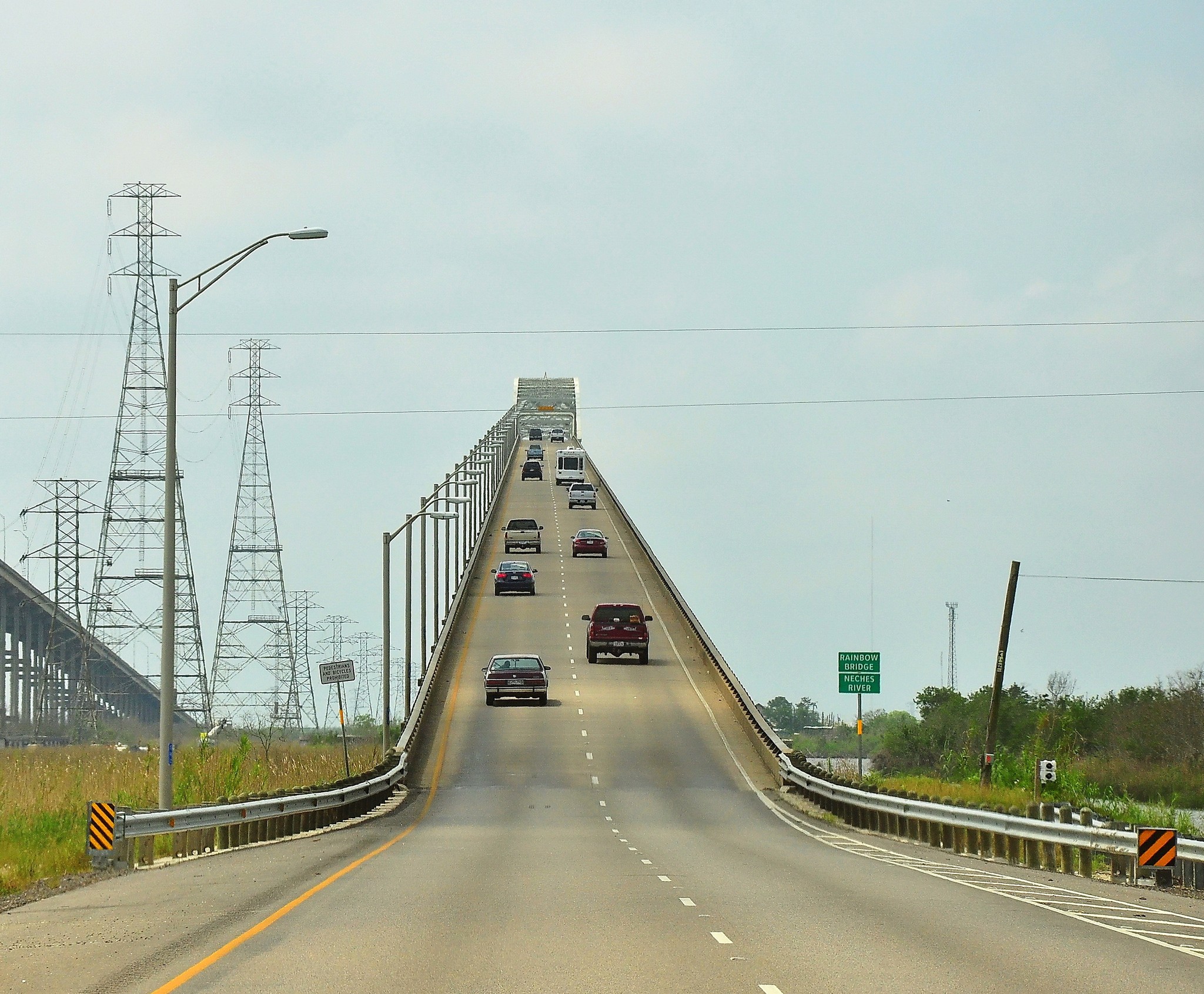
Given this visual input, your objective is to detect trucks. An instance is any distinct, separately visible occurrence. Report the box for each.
[555,446,587,486]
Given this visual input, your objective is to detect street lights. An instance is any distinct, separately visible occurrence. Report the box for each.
[157,224,331,814]
[381,404,521,764]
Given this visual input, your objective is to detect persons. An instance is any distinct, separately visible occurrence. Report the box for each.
[504,661,511,668]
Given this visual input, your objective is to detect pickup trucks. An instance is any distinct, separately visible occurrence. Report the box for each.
[524,444,546,461]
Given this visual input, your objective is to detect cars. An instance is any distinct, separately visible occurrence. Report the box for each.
[529,428,544,441]
[481,653,552,706]
[519,460,545,481]
[566,482,598,510]
[549,428,566,443]
[581,603,653,665]
[490,560,538,596]
[500,518,544,554]
